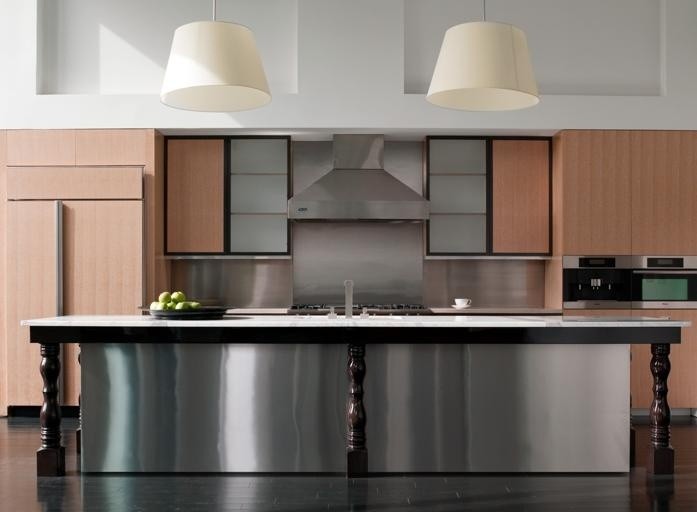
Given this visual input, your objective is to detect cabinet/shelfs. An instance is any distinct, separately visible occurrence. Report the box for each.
[0,128,172,417]
[423,135,552,261]
[163,136,293,261]
[544,130,695,409]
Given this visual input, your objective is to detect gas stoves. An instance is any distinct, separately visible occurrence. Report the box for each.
[287,300,433,315]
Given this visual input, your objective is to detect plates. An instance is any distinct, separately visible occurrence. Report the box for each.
[138,304,234,319]
[451,304,472,309]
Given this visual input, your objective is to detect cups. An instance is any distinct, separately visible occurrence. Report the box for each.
[455,298,472,305]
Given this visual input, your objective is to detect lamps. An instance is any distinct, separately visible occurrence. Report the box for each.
[161,1,272,115]
[424,1,540,113]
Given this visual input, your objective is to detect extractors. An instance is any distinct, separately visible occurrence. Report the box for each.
[287,132,431,227]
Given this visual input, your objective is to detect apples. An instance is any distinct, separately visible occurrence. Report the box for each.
[149,291,202,310]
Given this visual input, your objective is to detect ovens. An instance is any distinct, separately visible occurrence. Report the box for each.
[562,255,697,309]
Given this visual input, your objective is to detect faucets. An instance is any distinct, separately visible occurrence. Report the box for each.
[343,278,354,319]
